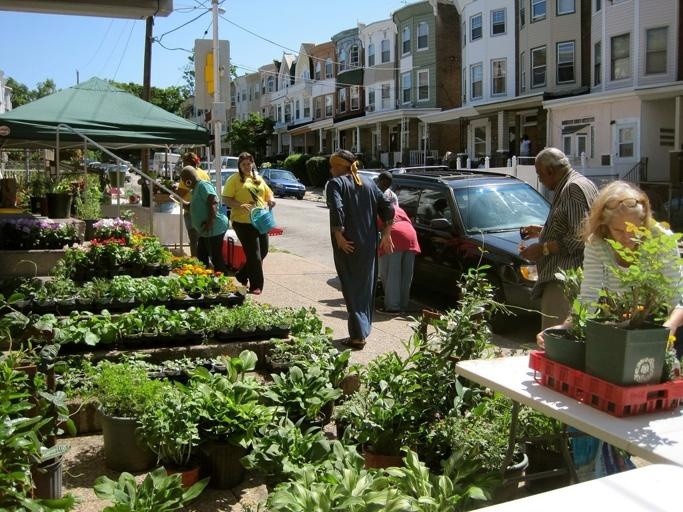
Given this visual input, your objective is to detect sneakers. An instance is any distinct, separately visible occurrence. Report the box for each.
[233,272,263,295]
[340,337,367,350]
[375,306,401,316]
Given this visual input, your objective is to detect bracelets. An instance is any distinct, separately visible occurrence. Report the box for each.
[536,178,683,367]
[542,242,549,256]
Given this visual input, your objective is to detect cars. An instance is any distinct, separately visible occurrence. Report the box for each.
[78,152,305,205]
[322,169,385,209]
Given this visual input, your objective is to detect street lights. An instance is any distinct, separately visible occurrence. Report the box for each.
[140,4,225,205]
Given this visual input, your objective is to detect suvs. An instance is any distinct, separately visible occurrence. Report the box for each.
[375,164,552,337]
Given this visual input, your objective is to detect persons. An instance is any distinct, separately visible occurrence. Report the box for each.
[414,198,448,225]
[325,148,392,349]
[372,188,420,319]
[518,147,598,335]
[519,134,532,164]
[362,172,391,203]
[220,150,276,295]
[373,177,399,207]
[174,151,210,255]
[179,166,225,275]
[470,191,503,227]
[477,158,484,168]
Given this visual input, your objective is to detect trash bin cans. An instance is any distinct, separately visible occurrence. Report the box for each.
[109,165,128,187]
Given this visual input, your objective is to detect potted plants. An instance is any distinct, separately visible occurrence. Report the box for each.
[1,168,175,218]
